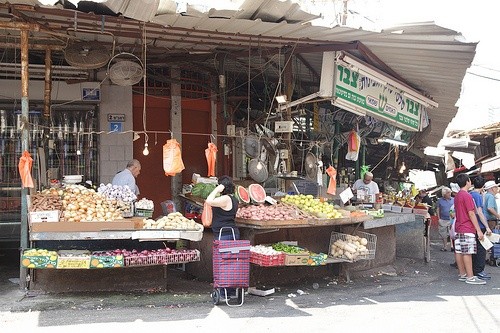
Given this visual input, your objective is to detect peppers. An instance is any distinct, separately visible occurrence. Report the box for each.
[272,242,305,253]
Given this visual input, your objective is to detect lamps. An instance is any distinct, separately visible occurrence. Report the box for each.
[377,138,408,147]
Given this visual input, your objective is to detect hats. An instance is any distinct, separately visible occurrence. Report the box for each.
[483,180,500,190]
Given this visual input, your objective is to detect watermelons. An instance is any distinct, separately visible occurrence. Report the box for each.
[235,184,267,206]
[191,182,217,199]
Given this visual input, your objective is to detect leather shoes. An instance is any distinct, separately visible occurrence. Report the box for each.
[475,272,492,280]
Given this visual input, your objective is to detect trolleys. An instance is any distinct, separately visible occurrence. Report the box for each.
[211,227,251,307]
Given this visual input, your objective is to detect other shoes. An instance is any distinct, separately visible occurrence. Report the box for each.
[450,248,455,252]
[441,248,447,252]
[450,262,458,269]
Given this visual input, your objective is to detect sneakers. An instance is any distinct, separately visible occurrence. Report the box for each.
[467,276,487,284]
[458,274,468,281]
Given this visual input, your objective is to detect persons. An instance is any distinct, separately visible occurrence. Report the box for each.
[352,171,380,203]
[437,187,455,251]
[110,158,141,249]
[468,176,491,279]
[483,180,500,264]
[454,173,487,285]
[419,190,435,237]
[450,182,500,269]
[206,176,240,298]
[396,189,439,216]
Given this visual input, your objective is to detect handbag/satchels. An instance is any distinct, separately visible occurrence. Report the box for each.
[200,201,213,229]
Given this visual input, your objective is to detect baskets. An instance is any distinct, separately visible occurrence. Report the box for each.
[125,249,200,268]
[328,231,377,262]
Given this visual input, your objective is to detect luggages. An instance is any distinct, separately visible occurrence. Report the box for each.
[490,219,500,267]
[211,227,250,307]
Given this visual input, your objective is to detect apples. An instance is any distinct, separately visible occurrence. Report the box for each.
[54,188,129,222]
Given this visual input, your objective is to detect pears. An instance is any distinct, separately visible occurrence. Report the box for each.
[281,194,345,219]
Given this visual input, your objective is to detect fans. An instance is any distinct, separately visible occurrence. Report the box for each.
[305,152,323,185]
[64,41,111,69]
[106,52,145,87]
[245,134,261,156]
[262,137,278,155]
[249,148,269,183]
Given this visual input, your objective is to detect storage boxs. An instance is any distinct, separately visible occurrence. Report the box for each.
[30,210,60,223]
[57,250,90,269]
[264,242,309,266]
[249,252,285,267]
[382,204,429,214]
[90,251,125,268]
[22,249,57,269]
[135,209,153,217]
[329,227,377,262]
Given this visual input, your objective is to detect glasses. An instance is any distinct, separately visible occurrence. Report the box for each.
[216,184,218,187]
